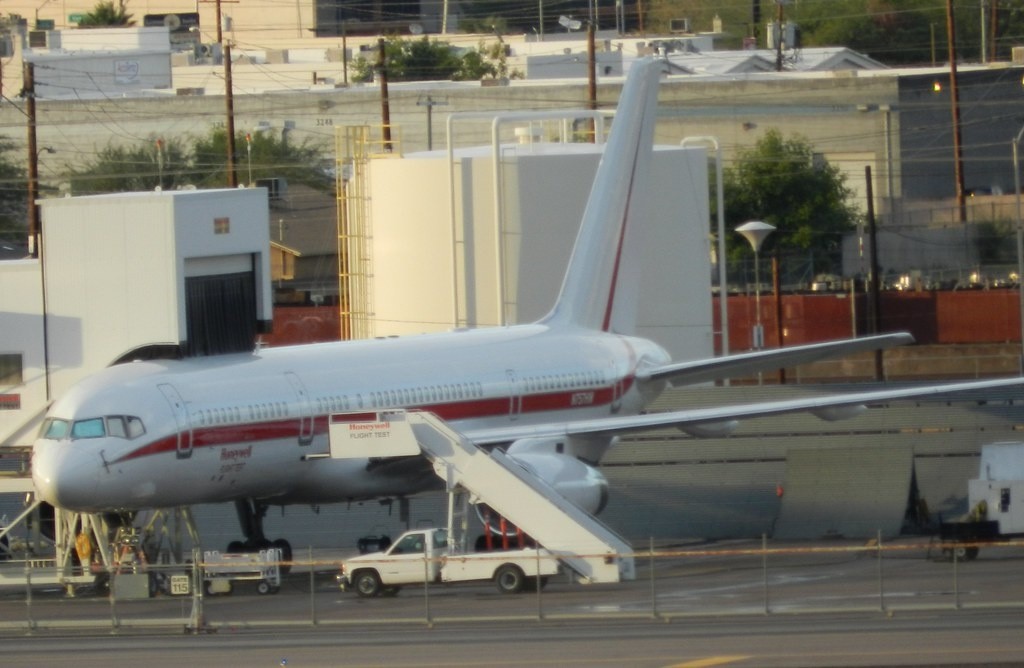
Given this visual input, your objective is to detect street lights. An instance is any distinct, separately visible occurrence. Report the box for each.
[733,221,777,385]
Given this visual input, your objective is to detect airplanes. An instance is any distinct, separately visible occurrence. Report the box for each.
[29,54,1024,584]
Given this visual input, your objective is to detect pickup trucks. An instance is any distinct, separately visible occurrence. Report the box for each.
[342,526,560,598]
